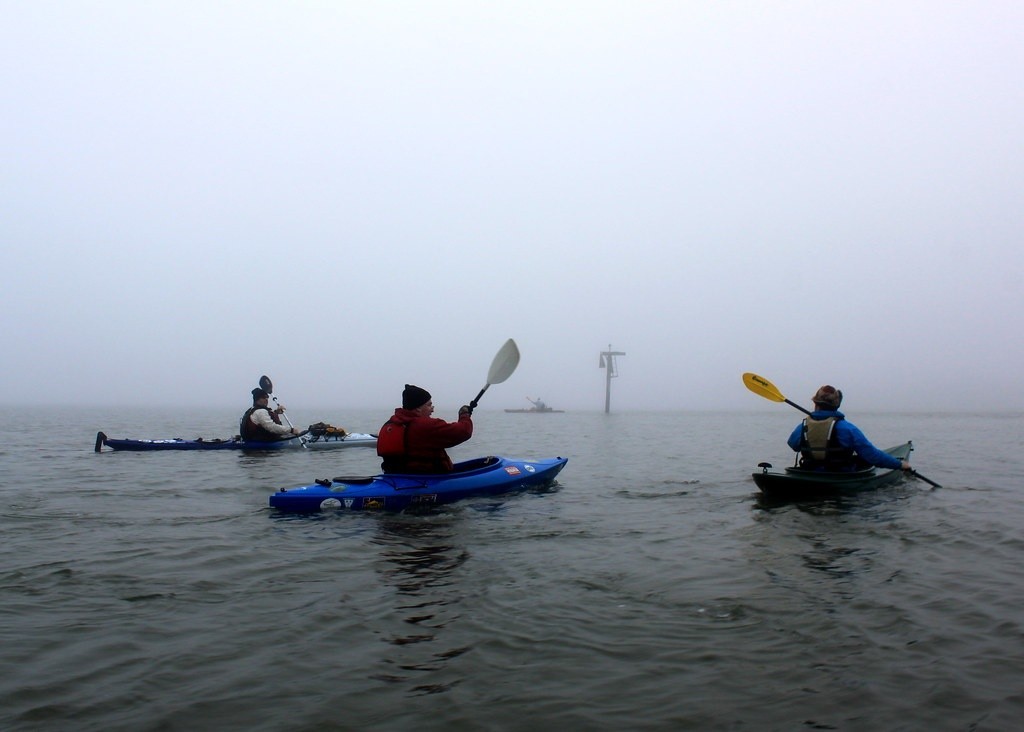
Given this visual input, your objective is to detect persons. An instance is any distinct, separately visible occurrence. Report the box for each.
[377,384,473,475]
[240,388,299,443]
[787,385,912,478]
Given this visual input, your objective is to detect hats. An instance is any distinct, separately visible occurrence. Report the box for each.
[251,388,267,402]
[402,384,432,411]
[811,385,843,407]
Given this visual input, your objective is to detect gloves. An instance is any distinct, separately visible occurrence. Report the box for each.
[459,405,473,418]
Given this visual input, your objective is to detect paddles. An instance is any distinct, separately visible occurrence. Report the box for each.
[259,375,308,448]
[742,373,943,488]
[470,338,520,408]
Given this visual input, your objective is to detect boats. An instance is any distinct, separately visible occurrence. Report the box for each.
[268,454,567,515]
[504,405,566,414]
[94,422,379,453]
[752,439,915,499]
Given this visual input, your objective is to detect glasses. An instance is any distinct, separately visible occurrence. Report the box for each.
[261,395,269,399]
[424,402,435,410]
